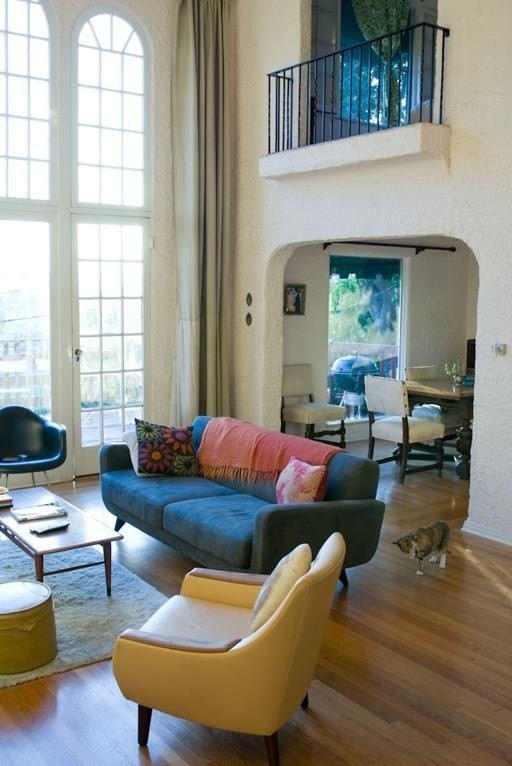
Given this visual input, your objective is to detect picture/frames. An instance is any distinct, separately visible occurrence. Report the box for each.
[284,284,307,316]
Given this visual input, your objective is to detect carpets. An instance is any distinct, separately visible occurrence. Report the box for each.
[0,539,170,689]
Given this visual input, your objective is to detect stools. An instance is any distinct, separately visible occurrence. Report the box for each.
[0,580,58,675]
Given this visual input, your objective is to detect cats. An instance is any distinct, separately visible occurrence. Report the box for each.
[392,520,452,576]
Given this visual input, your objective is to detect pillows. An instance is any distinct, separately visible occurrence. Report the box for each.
[121,417,203,478]
[249,543,313,634]
[275,455,329,504]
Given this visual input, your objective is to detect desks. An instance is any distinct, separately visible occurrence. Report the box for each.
[404,378,475,480]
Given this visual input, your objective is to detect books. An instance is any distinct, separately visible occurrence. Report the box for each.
[1,485,13,510]
[8,499,68,523]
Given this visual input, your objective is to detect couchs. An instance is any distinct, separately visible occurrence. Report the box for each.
[99,416,386,588]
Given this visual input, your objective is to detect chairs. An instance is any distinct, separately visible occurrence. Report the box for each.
[113,532,347,765]
[281,364,346,449]
[0,406,67,491]
[363,365,458,483]
